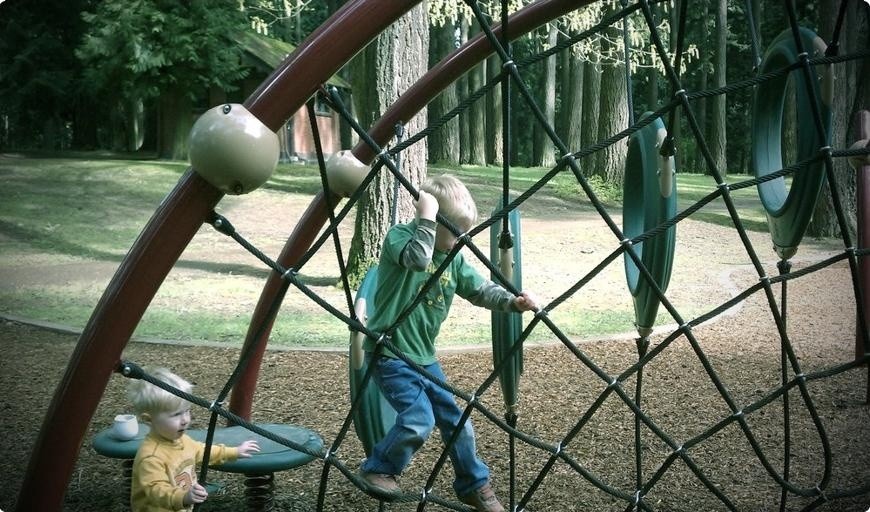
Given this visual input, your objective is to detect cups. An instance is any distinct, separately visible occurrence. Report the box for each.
[113,414,139,441]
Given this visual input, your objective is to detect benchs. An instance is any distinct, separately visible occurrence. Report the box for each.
[92,411,323,511]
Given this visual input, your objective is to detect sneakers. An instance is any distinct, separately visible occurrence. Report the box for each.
[358,470,404,500]
[456,483,505,512]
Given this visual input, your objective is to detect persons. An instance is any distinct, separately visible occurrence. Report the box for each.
[361,173,534,511]
[128,369,261,512]
